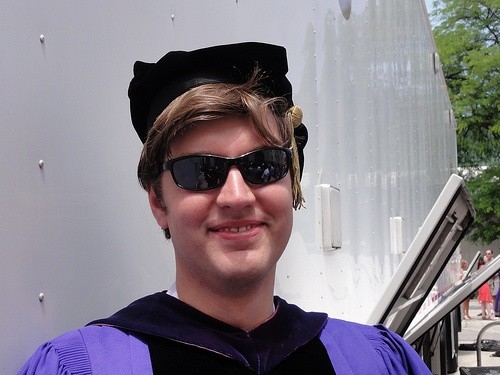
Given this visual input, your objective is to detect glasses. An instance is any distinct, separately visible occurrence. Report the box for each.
[156,147,292,192]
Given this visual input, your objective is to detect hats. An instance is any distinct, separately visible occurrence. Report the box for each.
[128,42,309,211]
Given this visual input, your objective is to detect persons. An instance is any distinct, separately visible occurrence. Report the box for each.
[17,41,434,375]
[461,250,500,320]
